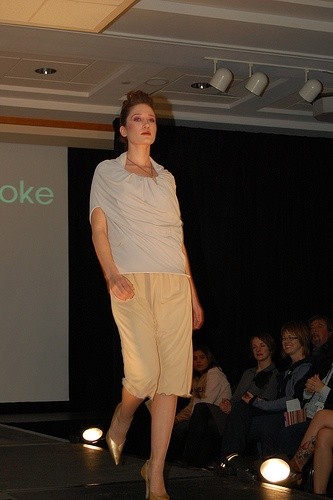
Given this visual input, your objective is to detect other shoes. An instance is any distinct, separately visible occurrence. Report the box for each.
[283,472,305,485]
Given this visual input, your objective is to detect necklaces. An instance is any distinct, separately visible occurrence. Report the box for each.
[128,158,158,186]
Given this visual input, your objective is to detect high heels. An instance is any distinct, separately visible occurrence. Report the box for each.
[140,460,151,500]
[106,401,133,465]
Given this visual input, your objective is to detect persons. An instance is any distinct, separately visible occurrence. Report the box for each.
[272,359,333,496]
[307,314,333,377]
[241,319,317,460]
[88,90,203,500]
[186,332,279,468]
[159,346,232,461]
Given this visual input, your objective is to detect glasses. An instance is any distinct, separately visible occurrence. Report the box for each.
[280,335,298,341]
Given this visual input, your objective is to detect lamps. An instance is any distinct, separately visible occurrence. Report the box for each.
[244,64,269,97]
[298,70,322,103]
[208,59,233,93]
